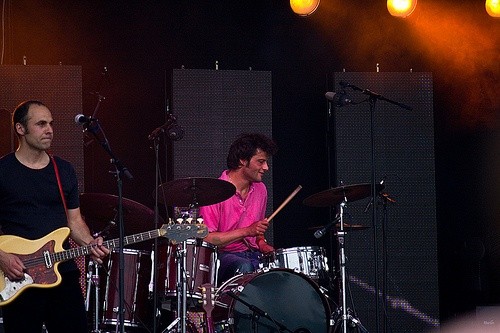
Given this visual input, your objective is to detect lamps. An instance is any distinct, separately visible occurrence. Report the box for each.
[289,0,320,17]
[386,0,418,17]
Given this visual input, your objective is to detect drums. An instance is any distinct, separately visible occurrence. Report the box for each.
[257,243,334,283]
[147,236,219,310]
[87,244,151,329]
[205,262,336,333]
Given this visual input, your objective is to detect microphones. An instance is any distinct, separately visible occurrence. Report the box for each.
[75,114,92,125]
[325,91,352,106]
[363,176,387,214]
[314,213,345,239]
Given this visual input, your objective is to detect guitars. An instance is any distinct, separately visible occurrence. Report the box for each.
[0,217,209,308]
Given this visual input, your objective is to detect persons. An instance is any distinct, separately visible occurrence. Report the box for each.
[195,132,274,285]
[0,100,110,333]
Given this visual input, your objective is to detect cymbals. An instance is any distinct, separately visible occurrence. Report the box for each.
[77,192,165,234]
[302,185,386,206]
[148,176,237,210]
[308,222,373,229]
